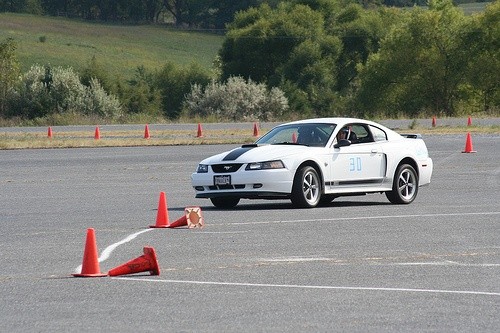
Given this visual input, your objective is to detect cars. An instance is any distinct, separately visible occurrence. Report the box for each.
[190,117,434,209]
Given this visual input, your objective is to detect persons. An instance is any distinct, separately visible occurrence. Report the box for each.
[337,127,351,141]
[298,128,314,143]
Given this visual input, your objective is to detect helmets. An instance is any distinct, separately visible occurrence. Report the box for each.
[336,127,351,141]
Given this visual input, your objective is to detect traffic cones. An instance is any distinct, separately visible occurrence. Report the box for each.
[150,192,170,229]
[69,228,107,278]
[197,124,205,138]
[432,116,437,127]
[292,133,297,143]
[47,127,52,138]
[170,206,204,229]
[467,117,473,128]
[144,124,150,139]
[461,131,477,153]
[94,127,100,139]
[253,124,259,136]
[109,246,161,277]
